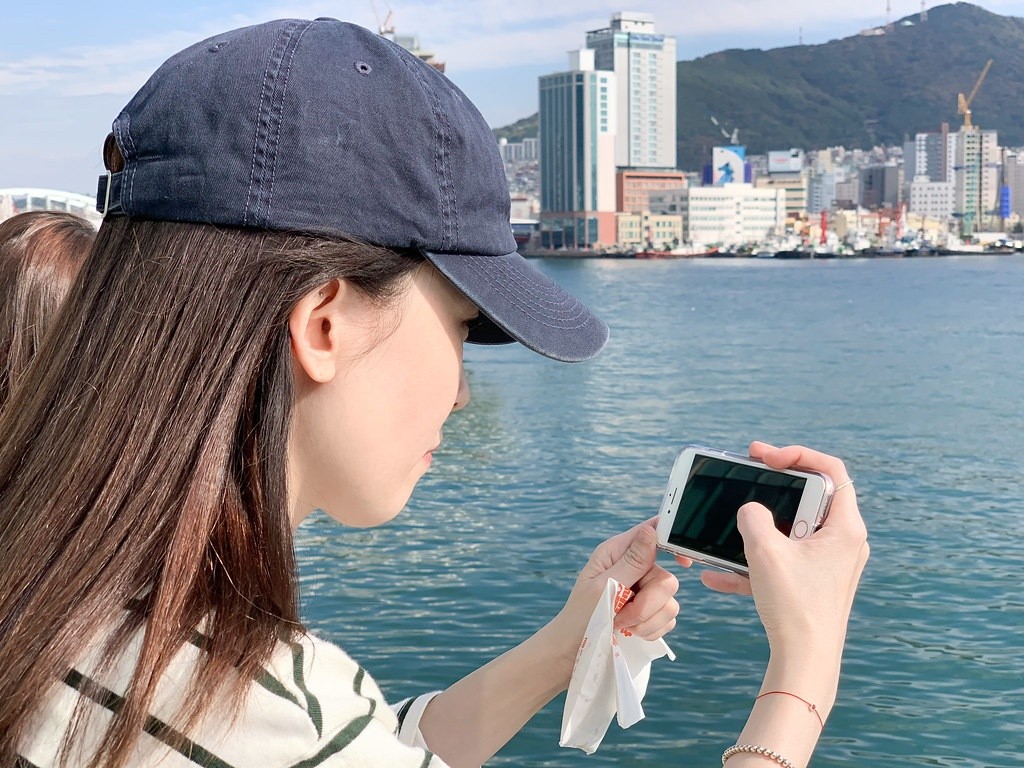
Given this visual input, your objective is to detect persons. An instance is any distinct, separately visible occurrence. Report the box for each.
[0,16,869,768]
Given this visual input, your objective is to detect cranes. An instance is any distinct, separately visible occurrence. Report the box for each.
[957,56,994,127]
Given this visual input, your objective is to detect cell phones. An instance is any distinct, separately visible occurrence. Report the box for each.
[652,444,833,580]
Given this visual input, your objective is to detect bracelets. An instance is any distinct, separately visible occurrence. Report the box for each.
[753,691,824,731]
[722,744,795,768]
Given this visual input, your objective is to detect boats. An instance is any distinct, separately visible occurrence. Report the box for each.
[636,241,718,257]
[775,250,833,259]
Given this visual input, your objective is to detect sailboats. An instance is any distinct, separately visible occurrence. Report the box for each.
[931,135,1015,256]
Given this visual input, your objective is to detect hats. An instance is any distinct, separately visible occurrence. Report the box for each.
[95,15,609,363]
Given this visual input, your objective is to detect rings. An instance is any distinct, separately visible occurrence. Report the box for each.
[836,481,854,492]
